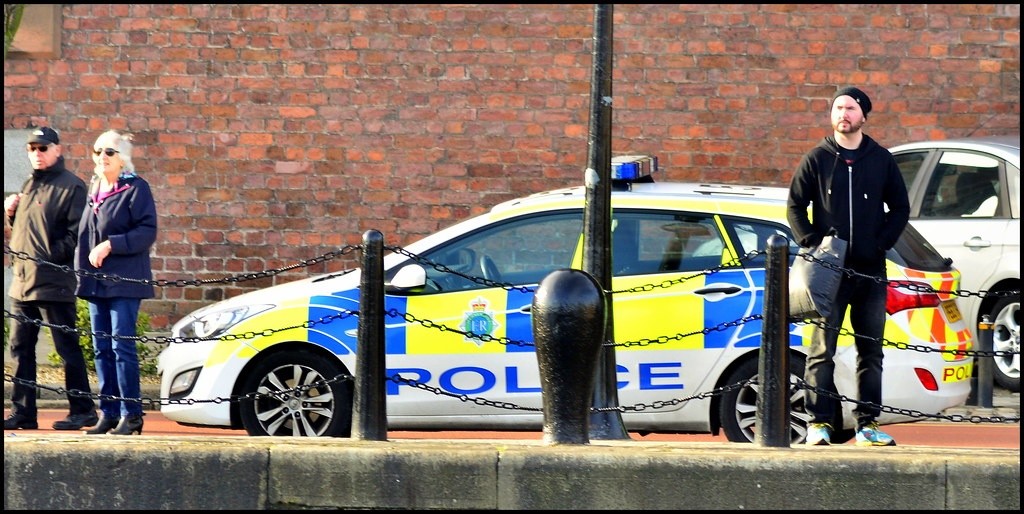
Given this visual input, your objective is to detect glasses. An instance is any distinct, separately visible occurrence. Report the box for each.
[92,148,116,158]
[26,145,54,152]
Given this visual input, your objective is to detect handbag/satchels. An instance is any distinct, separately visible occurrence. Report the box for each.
[789,235,848,318]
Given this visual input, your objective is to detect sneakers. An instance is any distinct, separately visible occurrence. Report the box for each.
[806,420,833,445]
[854,421,898,446]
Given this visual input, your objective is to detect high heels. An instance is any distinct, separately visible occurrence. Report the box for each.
[87,417,144,435]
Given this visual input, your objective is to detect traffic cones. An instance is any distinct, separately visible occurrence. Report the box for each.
[978,312,996,408]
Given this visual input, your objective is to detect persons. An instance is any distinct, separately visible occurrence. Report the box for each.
[4,127,157,436]
[787,87,911,445]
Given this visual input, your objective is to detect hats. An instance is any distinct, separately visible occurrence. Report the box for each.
[25,127,59,146]
[831,87,872,120]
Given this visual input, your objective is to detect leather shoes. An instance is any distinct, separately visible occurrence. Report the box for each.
[3,409,39,430]
[52,408,99,430]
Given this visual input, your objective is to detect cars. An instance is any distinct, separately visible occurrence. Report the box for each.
[885,135,1020,393]
[157,152,973,451]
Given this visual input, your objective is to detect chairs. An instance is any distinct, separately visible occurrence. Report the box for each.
[613,220,636,276]
[952,172,997,217]
